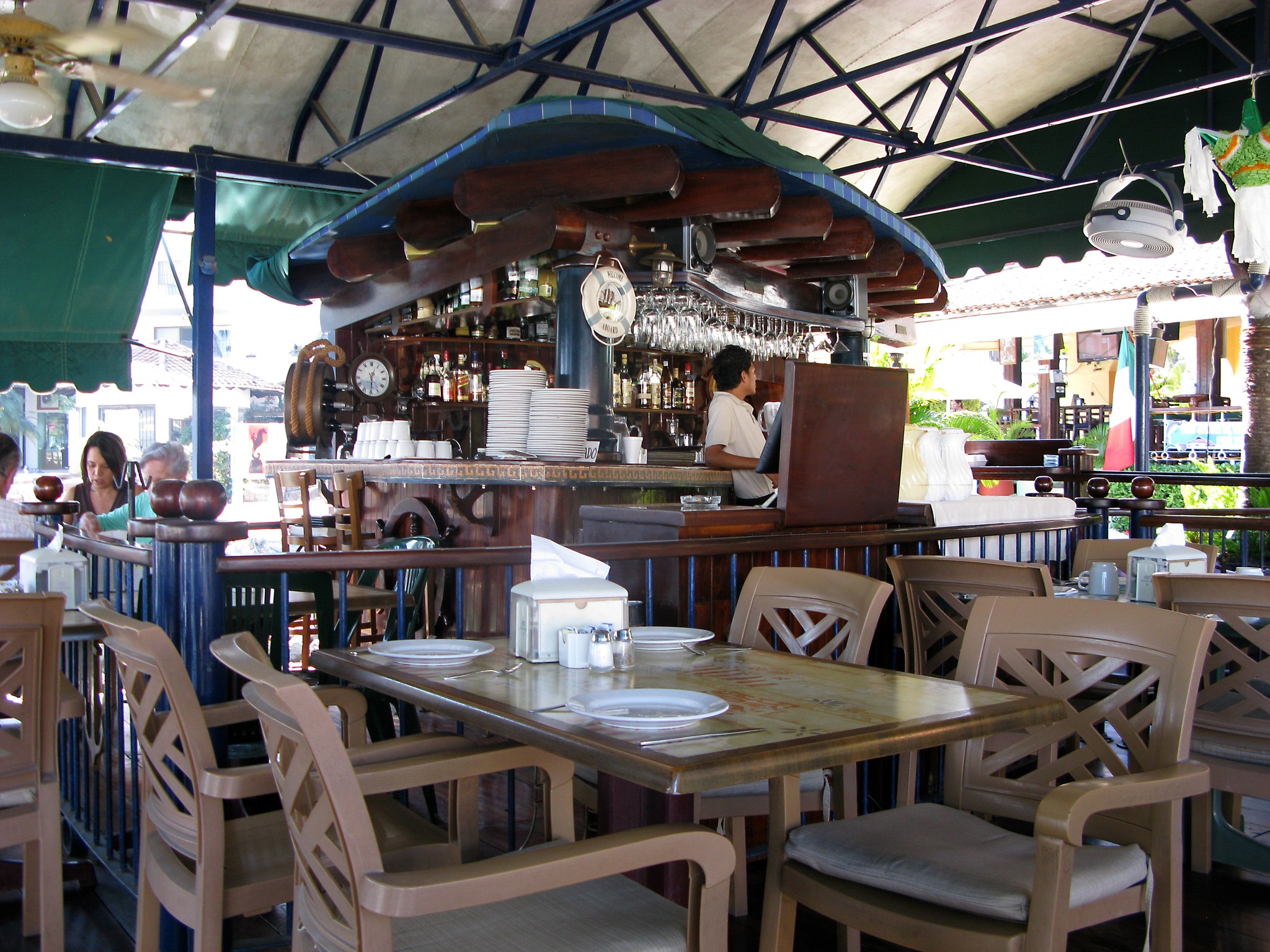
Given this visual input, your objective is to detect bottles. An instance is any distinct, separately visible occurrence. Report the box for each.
[411,351,509,403]
[321,378,354,432]
[502,263,557,301]
[427,312,557,343]
[588,628,635,672]
[474,448,492,460]
[2,585,25,594]
[612,354,695,410]
[531,367,554,388]
[434,286,483,315]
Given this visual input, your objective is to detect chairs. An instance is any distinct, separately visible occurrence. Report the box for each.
[0,539,1270,952]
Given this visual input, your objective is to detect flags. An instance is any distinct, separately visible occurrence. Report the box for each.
[1101,327,1136,471]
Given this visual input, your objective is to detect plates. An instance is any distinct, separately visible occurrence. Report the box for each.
[565,688,730,729]
[628,627,715,650]
[1053,576,1126,596]
[647,451,695,466]
[486,370,591,460]
[367,639,494,665]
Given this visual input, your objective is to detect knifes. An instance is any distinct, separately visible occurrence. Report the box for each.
[637,728,767,745]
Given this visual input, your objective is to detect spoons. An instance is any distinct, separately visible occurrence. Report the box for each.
[443,663,523,680]
[1051,578,1077,585]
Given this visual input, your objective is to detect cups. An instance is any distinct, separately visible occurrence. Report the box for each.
[622,437,643,463]
[353,421,453,460]
[757,404,781,435]
[1078,562,1120,595]
[1235,567,1264,576]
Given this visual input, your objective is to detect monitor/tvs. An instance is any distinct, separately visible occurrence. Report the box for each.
[1076,330,1121,362]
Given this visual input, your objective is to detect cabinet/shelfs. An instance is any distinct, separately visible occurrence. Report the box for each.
[382,336,701,460]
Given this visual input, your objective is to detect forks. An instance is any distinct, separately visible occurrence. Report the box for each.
[679,643,754,655]
[350,635,436,654]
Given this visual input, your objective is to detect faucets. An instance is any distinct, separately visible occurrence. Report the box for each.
[628,425,642,437]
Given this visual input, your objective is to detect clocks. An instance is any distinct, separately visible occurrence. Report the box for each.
[344,342,405,413]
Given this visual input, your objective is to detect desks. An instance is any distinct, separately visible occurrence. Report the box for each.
[311,638,1068,952]
[1173,394,1209,422]
[225,579,418,672]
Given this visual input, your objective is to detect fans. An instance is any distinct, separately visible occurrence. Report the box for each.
[0,0,218,129]
[1083,170,1187,259]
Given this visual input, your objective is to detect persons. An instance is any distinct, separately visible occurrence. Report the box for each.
[951,399,969,411]
[704,344,779,509]
[80,440,191,623]
[0,433,42,586]
[60,430,143,531]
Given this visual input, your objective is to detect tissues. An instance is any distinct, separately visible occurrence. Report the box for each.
[1124,522,1207,603]
[509,533,628,667]
[18,522,92,611]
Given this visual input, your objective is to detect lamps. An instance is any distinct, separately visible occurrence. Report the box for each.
[630,235,686,288]
[0,82,57,129]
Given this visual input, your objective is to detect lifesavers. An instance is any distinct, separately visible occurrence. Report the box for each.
[581,264,637,339]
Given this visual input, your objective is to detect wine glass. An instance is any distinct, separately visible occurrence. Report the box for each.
[394,397,413,428]
[631,285,850,361]
[337,427,358,460]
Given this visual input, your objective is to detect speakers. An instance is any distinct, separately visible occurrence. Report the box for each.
[656,222,716,276]
[820,271,868,321]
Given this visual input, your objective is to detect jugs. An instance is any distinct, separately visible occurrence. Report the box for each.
[898,423,974,501]
[613,414,643,453]
